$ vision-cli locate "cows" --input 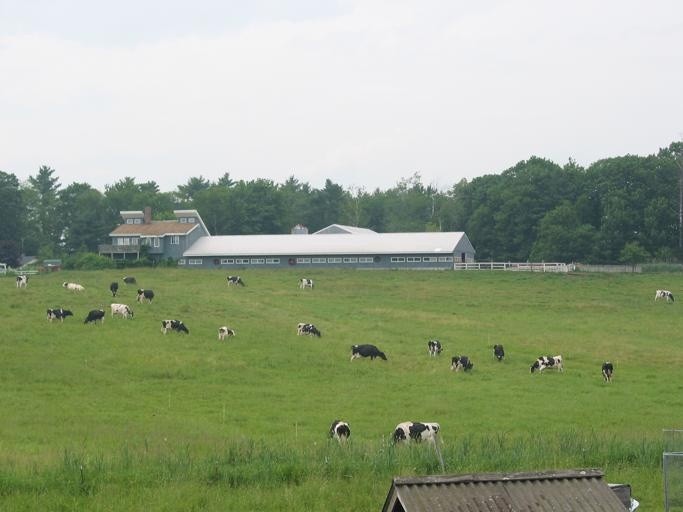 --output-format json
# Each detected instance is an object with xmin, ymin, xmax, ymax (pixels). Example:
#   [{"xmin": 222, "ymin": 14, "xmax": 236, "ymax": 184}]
[
  {"xmin": 161, "ymin": 319, "xmax": 190, "ymax": 337},
  {"xmin": 298, "ymin": 277, "xmax": 315, "ymax": 291},
  {"xmin": 297, "ymin": 322, "xmax": 320, "ymax": 338},
  {"xmin": 45, "ymin": 276, "xmax": 155, "ymax": 326},
  {"xmin": 16, "ymin": 275, "xmax": 28, "ymax": 288},
  {"xmin": 655, "ymin": 289, "xmax": 675, "ymax": 304},
  {"xmin": 327, "ymin": 420, "xmax": 352, "ymax": 443},
  {"xmin": 218, "ymin": 327, "xmax": 237, "ymax": 341},
  {"xmin": 602, "ymin": 361, "xmax": 615, "ymax": 384},
  {"xmin": 427, "ymin": 339, "xmax": 474, "ymax": 373},
  {"xmin": 390, "ymin": 420, "xmax": 444, "ymax": 453},
  {"xmin": 350, "ymin": 344, "xmax": 387, "ymax": 361},
  {"xmin": 530, "ymin": 354, "xmax": 564, "ymax": 375},
  {"xmin": 493, "ymin": 345, "xmax": 505, "ymax": 362},
  {"xmin": 226, "ymin": 276, "xmax": 245, "ymax": 287}
]
